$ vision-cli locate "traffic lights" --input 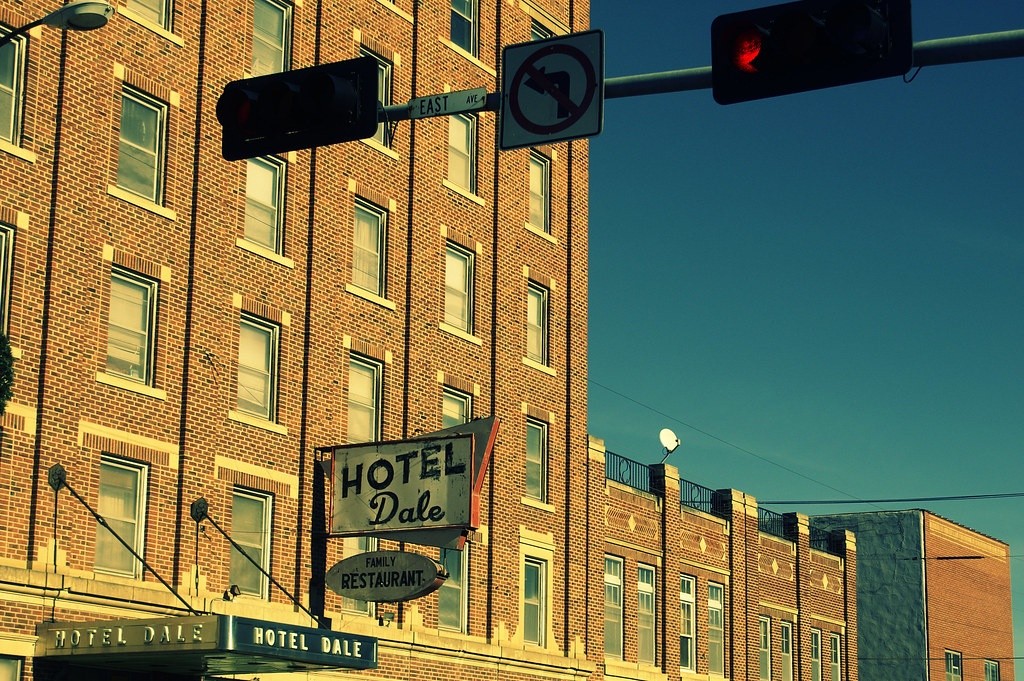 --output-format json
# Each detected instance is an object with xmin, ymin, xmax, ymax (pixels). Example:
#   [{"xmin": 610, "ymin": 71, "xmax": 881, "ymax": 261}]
[
  {"xmin": 215, "ymin": 55, "xmax": 380, "ymax": 162},
  {"xmin": 711, "ymin": 0, "xmax": 914, "ymax": 107}
]
